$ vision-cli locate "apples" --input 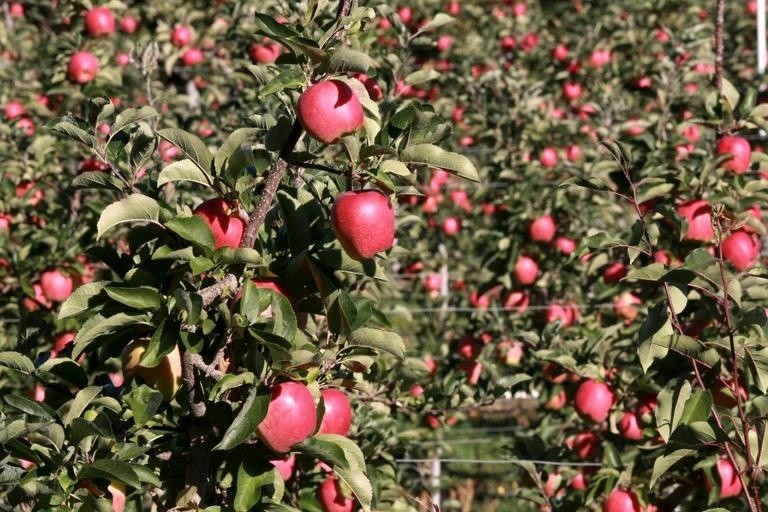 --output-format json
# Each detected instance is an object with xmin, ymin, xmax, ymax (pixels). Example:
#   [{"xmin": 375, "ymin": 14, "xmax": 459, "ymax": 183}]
[{"xmin": 0, "ymin": 0, "xmax": 768, "ymax": 512}]
